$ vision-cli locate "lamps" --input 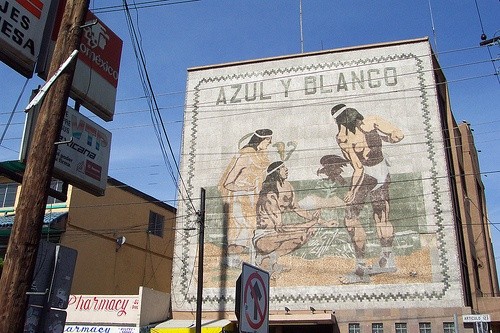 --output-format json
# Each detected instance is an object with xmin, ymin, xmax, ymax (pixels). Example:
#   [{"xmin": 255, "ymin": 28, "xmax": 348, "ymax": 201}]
[{"xmin": 115, "ymin": 235, "xmax": 126, "ymax": 253}]
